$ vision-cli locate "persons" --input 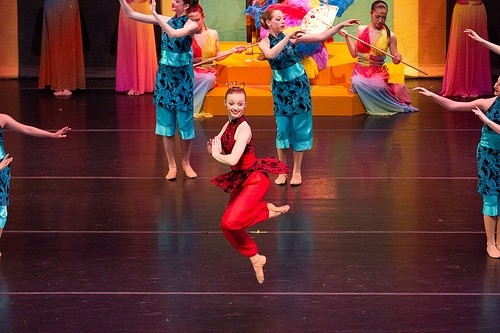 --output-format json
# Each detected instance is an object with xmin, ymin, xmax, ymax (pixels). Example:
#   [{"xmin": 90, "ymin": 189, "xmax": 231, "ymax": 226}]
[
  {"xmin": 38, "ymin": 1, "xmax": 86, "ymax": 97},
  {"xmin": 258, "ymin": 10, "xmax": 360, "ymax": 185},
  {"xmin": 115, "ymin": 1, "xmax": 159, "ymax": 96},
  {"xmin": 118, "ymin": 0, "xmax": 199, "ymax": 180},
  {"xmin": 338, "ymin": 0, "xmax": 404, "ymax": 116},
  {"xmin": 0, "ymin": 109, "xmax": 72, "ymax": 258},
  {"xmin": 206, "ymin": 87, "xmax": 290, "ymax": 284},
  {"xmin": 184, "ymin": 3, "xmax": 247, "ymax": 119},
  {"xmin": 412, "ymin": 0, "xmax": 500, "ymax": 259}
]
